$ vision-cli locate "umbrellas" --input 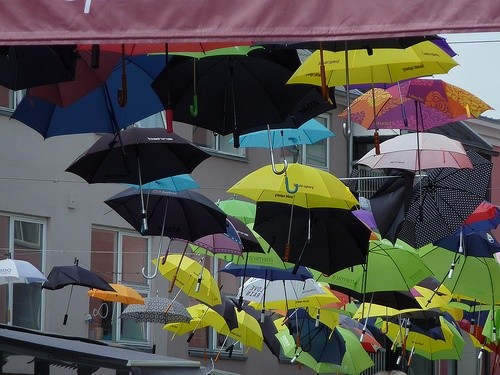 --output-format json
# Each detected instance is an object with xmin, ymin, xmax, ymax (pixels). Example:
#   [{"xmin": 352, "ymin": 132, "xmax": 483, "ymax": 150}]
[
  {"xmin": 64, "ymin": 124, "xmax": 214, "ymax": 230},
  {"xmin": 337, "ymin": 78, "xmax": 496, "ymax": 176},
  {"xmin": 225, "ymin": 160, "xmax": 362, "ymax": 262},
  {"xmin": 9, "ymin": 55, "xmax": 176, "ymax": 162},
  {"xmin": 151, "ymin": 44, "xmax": 338, "ymax": 149},
  {"xmin": 286, "ymin": 40, "xmax": 463, "ymax": 155},
  {"xmin": 0, "ymin": 132, "xmax": 500, "ymax": 375},
  {"xmin": 0, "ymin": 33, "xmax": 500, "ymax": 91},
  {"xmin": 225, "ymin": 118, "xmax": 336, "ymax": 175}
]
